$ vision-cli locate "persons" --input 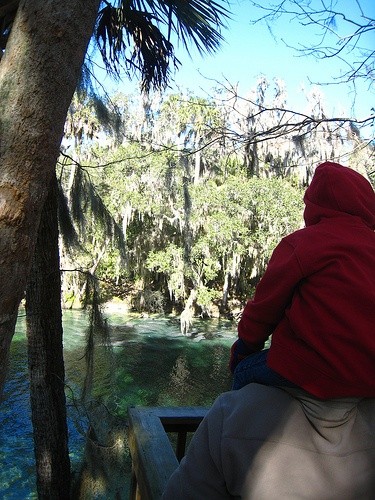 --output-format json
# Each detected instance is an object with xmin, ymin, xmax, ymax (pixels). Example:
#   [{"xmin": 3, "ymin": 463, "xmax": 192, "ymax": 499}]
[
  {"xmin": 226, "ymin": 159, "xmax": 374, "ymax": 401},
  {"xmin": 156, "ymin": 380, "xmax": 374, "ymax": 500}
]
